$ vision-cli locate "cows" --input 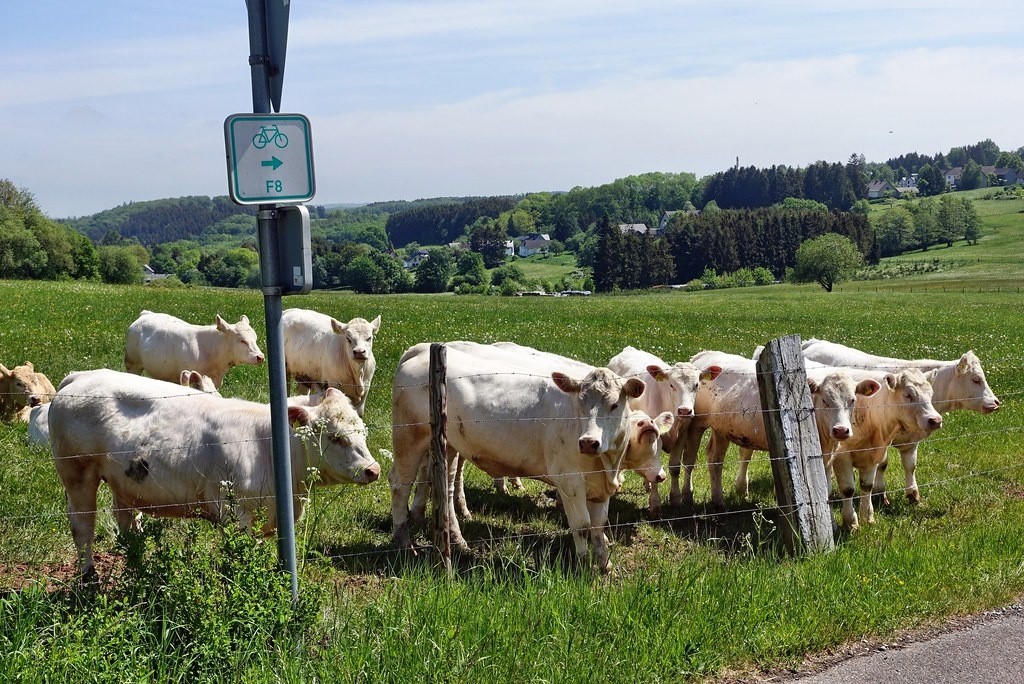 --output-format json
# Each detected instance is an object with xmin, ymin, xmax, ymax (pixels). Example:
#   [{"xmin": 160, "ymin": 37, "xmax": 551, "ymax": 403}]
[
  {"xmin": 0, "ymin": 358, "xmax": 57, "ymax": 425},
  {"xmin": 689, "ymin": 349, "xmax": 881, "ymax": 505},
  {"xmin": 750, "ymin": 345, "xmax": 944, "ymax": 535},
  {"xmin": 800, "ymin": 338, "xmax": 1001, "ymax": 504},
  {"xmin": 47, "ymin": 368, "xmax": 382, "ymax": 586},
  {"xmin": 123, "ymin": 309, "xmax": 266, "ymax": 391},
  {"xmin": 606, "ymin": 344, "xmax": 723, "ymax": 506},
  {"xmin": 490, "ymin": 408, "xmax": 674, "ymax": 528},
  {"xmin": 282, "ymin": 307, "xmax": 382, "ymax": 419},
  {"xmin": 391, "ymin": 341, "xmax": 646, "ymax": 569}
]
